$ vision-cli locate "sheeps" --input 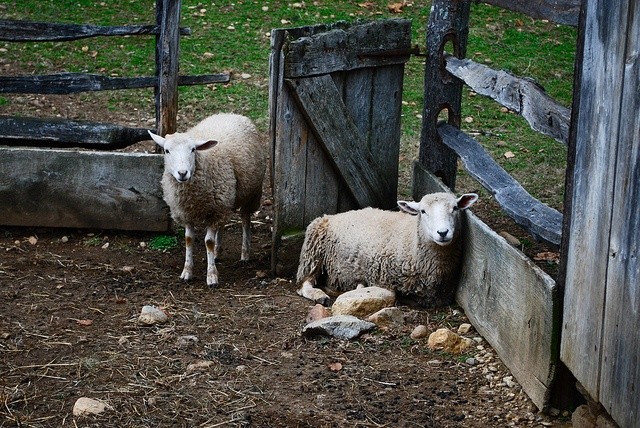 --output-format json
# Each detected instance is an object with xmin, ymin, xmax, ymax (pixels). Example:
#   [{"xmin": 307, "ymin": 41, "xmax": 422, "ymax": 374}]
[
  {"xmin": 147, "ymin": 112, "xmax": 267, "ymax": 290},
  {"xmin": 294, "ymin": 191, "xmax": 479, "ymax": 306}
]
